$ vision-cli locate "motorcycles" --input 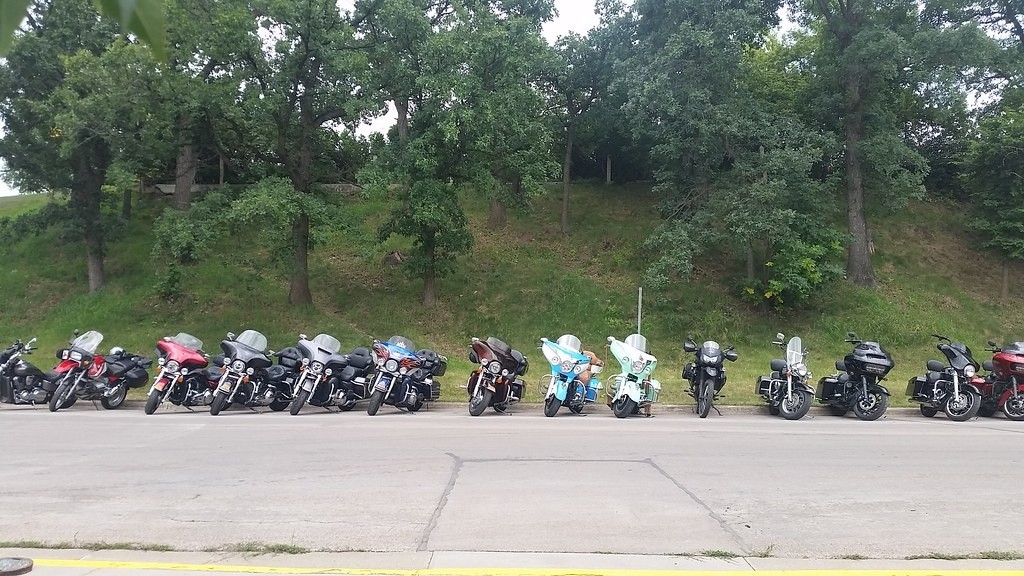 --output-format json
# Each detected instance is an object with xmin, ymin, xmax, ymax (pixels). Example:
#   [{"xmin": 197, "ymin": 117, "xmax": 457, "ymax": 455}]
[
  {"xmin": 966, "ymin": 341, "xmax": 1024, "ymax": 421},
  {"xmin": 209, "ymin": 329, "xmax": 309, "ymax": 415},
  {"xmin": 367, "ymin": 336, "xmax": 448, "ymax": 415},
  {"xmin": 143, "ymin": 332, "xmax": 257, "ymax": 414},
  {"xmin": 460, "ymin": 336, "xmax": 529, "ymax": 417},
  {"xmin": 289, "ymin": 333, "xmax": 381, "ymax": 414},
  {"xmin": 682, "ymin": 335, "xmax": 738, "ymax": 418},
  {"xmin": 605, "ymin": 334, "xmax": 661, "ymax": 418},
  {"xmin": 537, "ymin": 334, "xmax": 603, "ymax": 417},
  {"xmin": 0, "ymin": 337, "xmax": 80, "ymax": 408},
  {"xmin": 48, "ymin": 329, "xmax": 153, "ymax": 412},
  {"xmin": 904, "ymin": 333, "xmax": 982, "ymax": 421},
  {"xmin": 816, "ymin": 332, "xmax": 895, "ymax": 421},
  {"xmin": 754, "ymin": 333, "xmax": 817, "ymax": 419}
]
